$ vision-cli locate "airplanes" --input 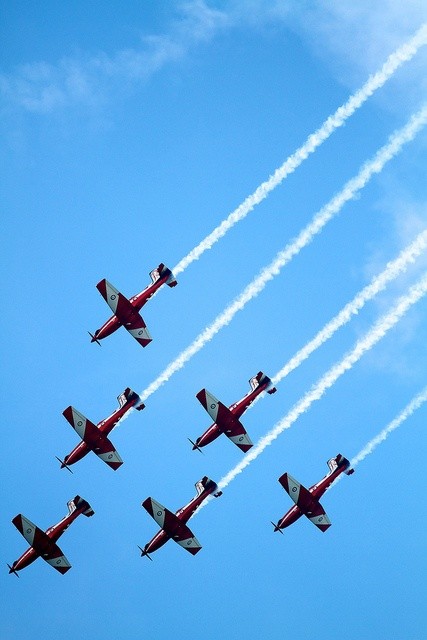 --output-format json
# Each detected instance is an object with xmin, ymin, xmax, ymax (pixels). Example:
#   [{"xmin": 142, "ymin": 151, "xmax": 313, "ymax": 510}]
[
  {"xmin": 5, "ymin": 495, "xmax": 94, "ymax": 580},
  {"xmin": 136, "ymin": 473, "xmax": 223, "ymax": 562},
  {"xmin": 86, "ymin": 261, "xmax": 178, "ymax": 349},
  {"xmin": 269, "ymin": 452, "xmax": 355, "ymax": 536},
  {"xmin": 186, "ymin": 368, "xmax": 278, "ymax": 456},
  {"xmin": 54, "ymin": 384, "xmax": 145, "ymax": 477}
]
